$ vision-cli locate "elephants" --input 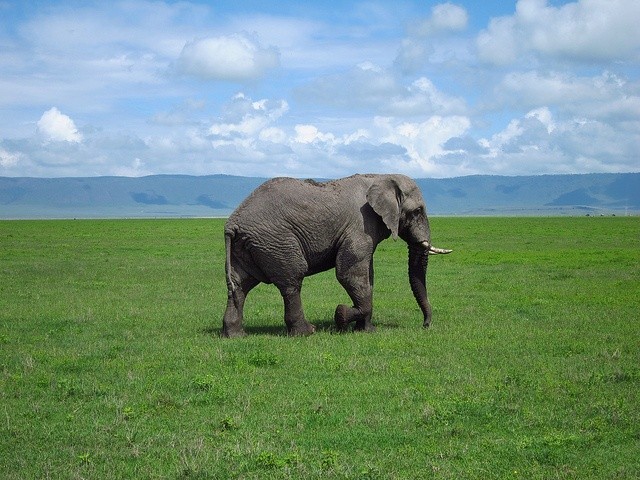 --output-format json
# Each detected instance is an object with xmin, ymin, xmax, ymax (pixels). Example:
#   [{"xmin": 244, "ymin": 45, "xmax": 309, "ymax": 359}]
[{"xmin": 222, "ymin": 173, "xmax": 453, "ymax": 339}]
